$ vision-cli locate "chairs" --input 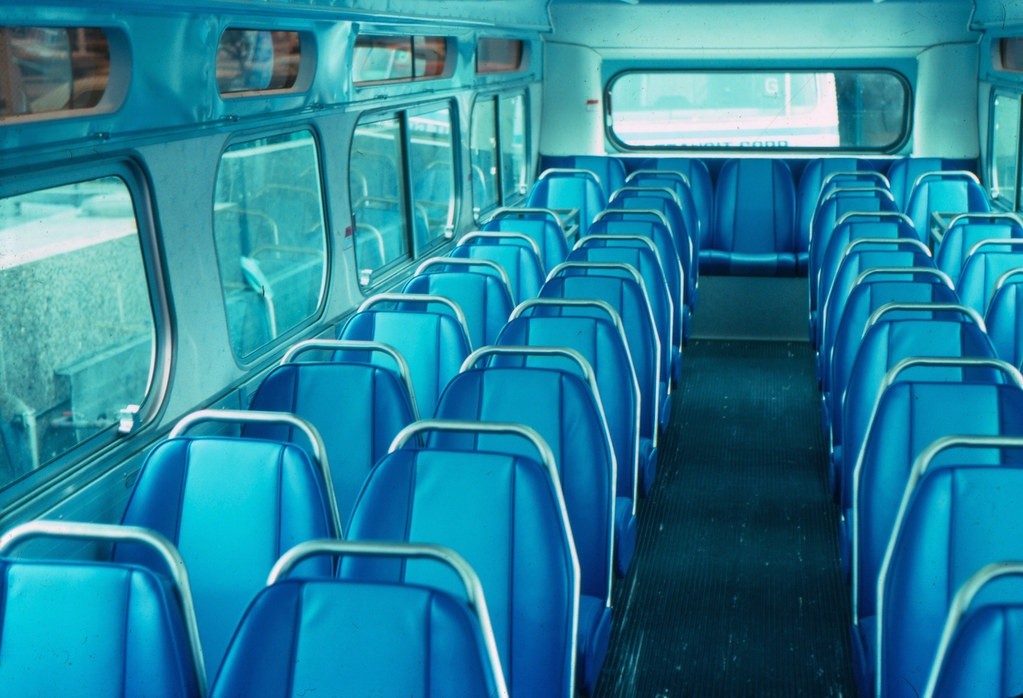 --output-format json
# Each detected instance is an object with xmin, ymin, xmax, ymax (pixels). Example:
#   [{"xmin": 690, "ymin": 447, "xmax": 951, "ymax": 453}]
[{"xmin": 0, "ymin": 148, "xmax": 1023, "ymax": 698}]
